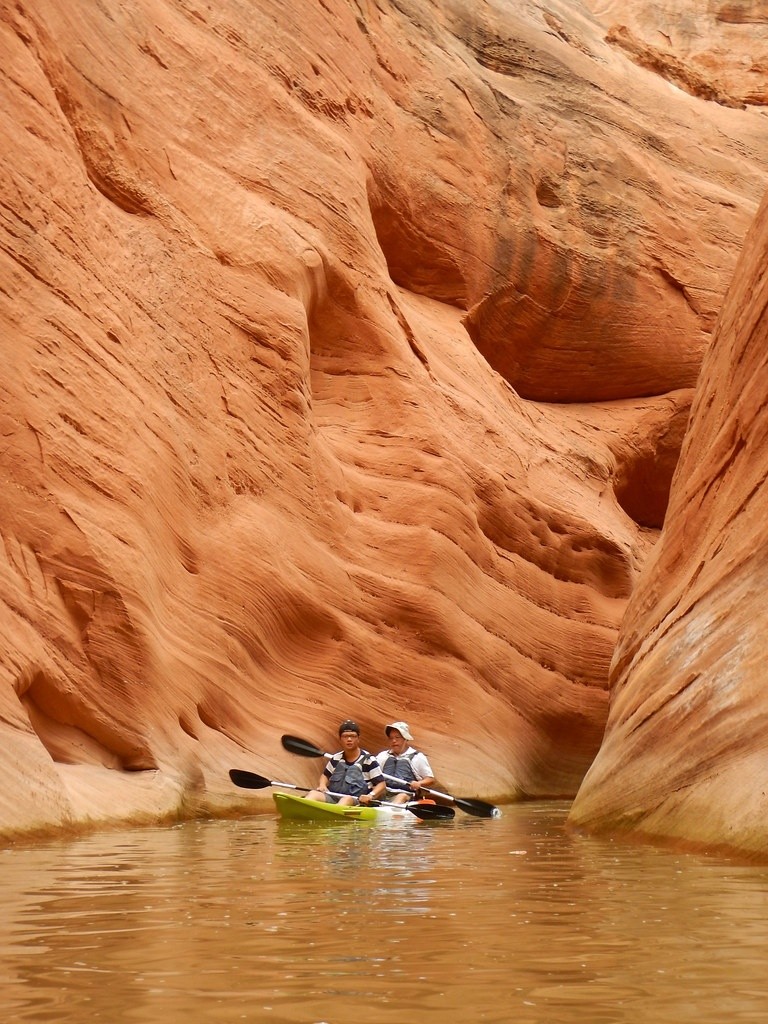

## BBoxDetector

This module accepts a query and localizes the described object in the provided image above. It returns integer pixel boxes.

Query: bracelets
[370,792,376,797]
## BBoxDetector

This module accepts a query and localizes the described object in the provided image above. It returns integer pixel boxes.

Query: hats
[385,722,414,740]
[339,720,359,736]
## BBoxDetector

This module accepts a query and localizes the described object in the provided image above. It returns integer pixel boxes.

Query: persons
[305,721,386,807]
[376,721,435,805]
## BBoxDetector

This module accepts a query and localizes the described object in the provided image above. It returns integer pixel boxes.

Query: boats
[271,790,437,822]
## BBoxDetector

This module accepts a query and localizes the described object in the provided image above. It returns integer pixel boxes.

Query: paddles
[228,768,456,821]
[280,734,503,819]
